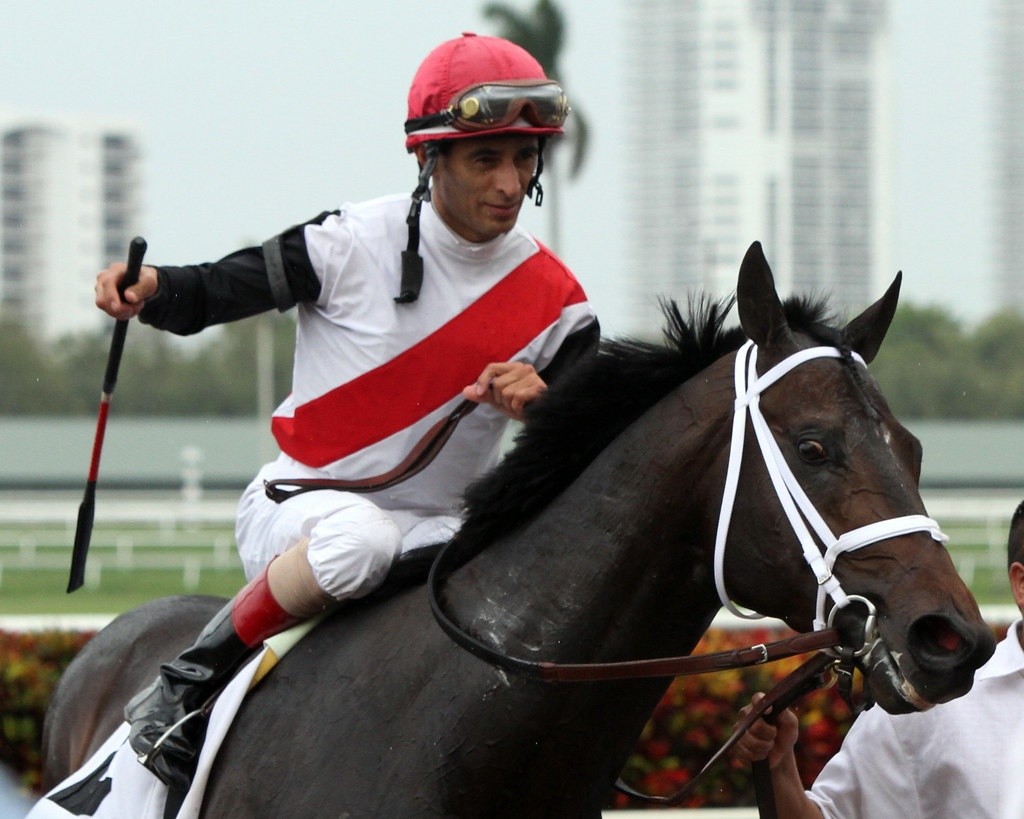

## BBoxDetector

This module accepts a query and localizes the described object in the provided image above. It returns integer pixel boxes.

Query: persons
[97,35,601,790]
[733,501,1024,819]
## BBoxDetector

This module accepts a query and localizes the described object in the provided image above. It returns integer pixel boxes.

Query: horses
[40,239,997,819]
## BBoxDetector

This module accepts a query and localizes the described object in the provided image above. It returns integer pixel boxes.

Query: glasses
[449,79,571,132]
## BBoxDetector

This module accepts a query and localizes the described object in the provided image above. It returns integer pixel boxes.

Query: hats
[404,31,574,155]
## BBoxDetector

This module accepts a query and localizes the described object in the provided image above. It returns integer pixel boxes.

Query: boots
[122,553,307,792]
